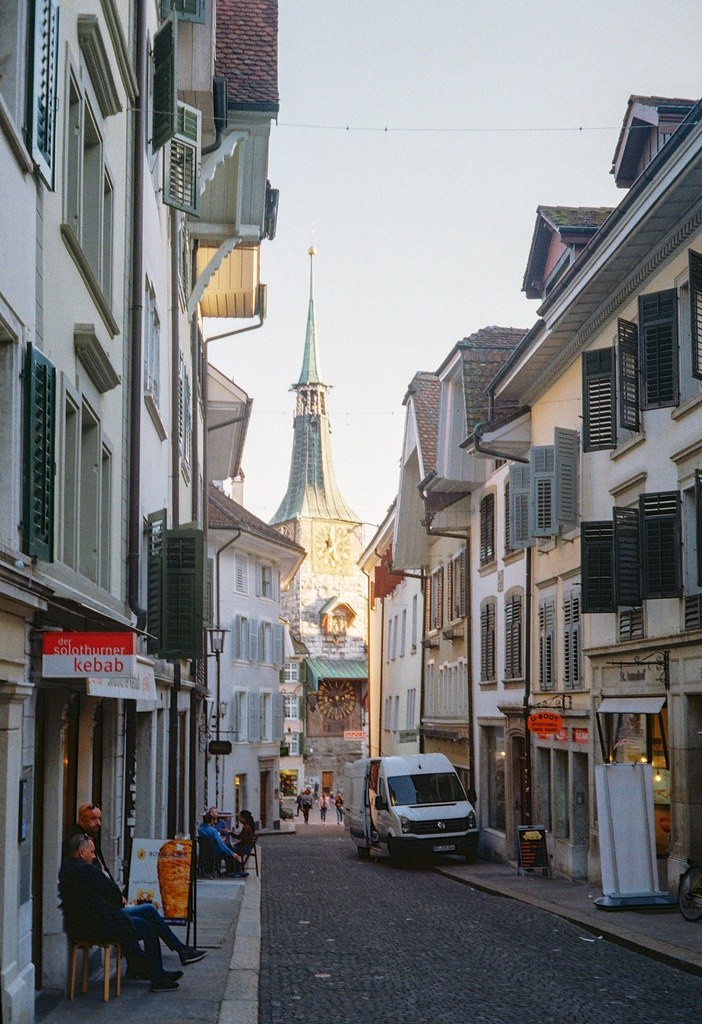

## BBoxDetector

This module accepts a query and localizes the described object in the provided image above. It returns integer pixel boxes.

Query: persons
[279,785,343,825]
[197,806,255,878]
[58,803,208,992]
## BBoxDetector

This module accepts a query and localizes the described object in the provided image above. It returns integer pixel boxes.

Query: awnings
[305,659,368,691]
[595,698,669,771]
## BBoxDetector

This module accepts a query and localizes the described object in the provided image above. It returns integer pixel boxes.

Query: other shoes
[163,970,184,982]
[150,974,180,992]
[179,946,208,966]
[234,870,249,878]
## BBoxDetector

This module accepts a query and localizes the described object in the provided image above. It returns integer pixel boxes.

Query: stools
[70,942,120,1000]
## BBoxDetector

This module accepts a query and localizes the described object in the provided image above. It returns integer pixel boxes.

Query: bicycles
[678,857,702,923]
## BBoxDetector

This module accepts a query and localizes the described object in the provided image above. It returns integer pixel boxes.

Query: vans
[346,753,479,867]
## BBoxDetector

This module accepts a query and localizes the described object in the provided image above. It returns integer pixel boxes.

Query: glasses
[76,833,95,850]
[80,802,101,814]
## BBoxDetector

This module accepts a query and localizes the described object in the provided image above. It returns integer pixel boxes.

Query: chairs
[198,839,222,879]
[240,832,260,876]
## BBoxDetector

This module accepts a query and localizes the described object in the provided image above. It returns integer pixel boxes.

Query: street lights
[209,628,230,807]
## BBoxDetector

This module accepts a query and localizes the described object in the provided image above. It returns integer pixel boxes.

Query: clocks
[318,680,356,720]
[314,524,352,568]
